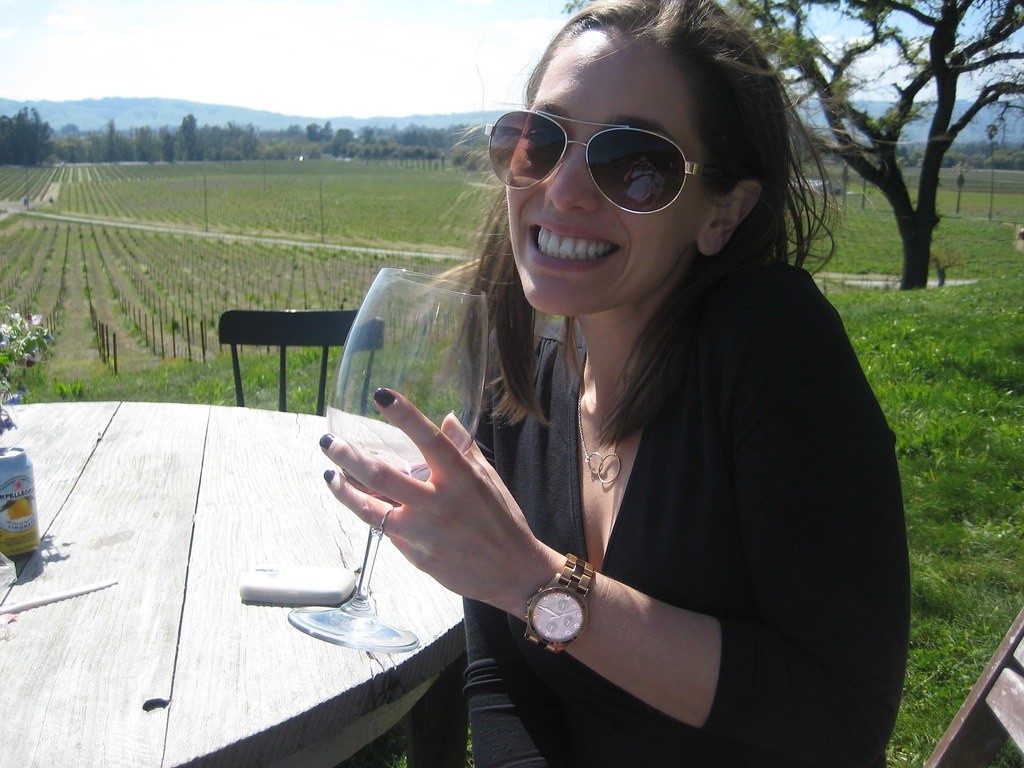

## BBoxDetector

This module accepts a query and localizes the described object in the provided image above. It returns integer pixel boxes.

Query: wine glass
[287,269,487,652]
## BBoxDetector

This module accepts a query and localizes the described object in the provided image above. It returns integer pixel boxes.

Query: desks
[0,400,465,768]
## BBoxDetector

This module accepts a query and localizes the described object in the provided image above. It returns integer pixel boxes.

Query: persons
[320,2,911,768]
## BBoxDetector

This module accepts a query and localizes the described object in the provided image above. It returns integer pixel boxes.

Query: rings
[372,504,394,540]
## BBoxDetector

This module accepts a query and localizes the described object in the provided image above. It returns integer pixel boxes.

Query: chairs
[922,606,1024,768]
[216,310,385,415]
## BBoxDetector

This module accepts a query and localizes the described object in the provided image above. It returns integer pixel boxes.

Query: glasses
[485,109,716,215]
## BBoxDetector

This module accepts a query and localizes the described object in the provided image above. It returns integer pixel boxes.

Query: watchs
[523,553,595,656]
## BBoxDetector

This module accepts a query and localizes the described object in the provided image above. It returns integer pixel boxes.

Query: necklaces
[578,384,622,484]
